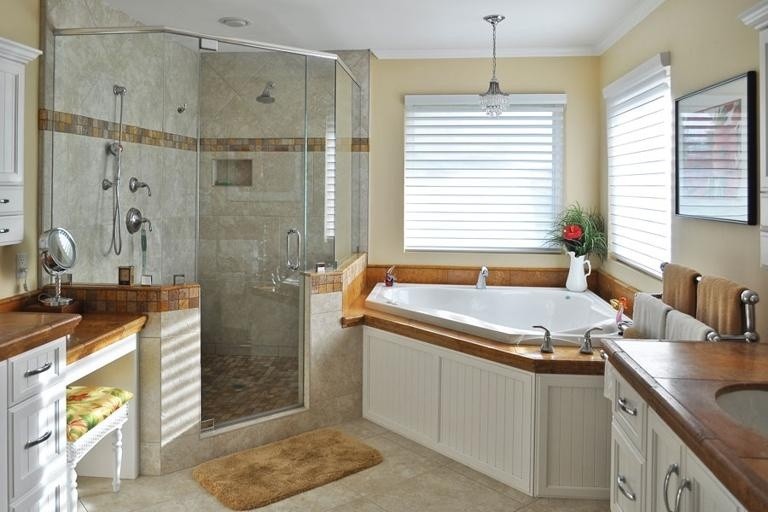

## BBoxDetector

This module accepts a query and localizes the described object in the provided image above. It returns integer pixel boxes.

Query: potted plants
[543,202,607,292]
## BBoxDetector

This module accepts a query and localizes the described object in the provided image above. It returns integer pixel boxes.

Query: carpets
[190,426,382,511]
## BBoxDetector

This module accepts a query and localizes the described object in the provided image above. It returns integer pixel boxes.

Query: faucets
[475,265,489,290]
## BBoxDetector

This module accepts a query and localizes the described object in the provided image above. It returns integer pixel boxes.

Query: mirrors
[38,227,77,307]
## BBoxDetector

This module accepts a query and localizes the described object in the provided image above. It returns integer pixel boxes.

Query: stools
[67,385,133,511]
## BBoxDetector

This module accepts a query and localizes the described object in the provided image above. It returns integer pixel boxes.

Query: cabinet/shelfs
[654,439,738,511]
[8,340,65,403]
[4,216,22,242]
[0,37,44,183]
[6,388,65,498]
[613,384,643,448]
[609,421,649,511]
[0,188,23,213]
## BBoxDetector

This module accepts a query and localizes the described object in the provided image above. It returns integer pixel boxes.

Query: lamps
[479,15,511,116]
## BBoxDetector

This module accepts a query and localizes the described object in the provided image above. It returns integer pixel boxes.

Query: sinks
[716,381,768,438]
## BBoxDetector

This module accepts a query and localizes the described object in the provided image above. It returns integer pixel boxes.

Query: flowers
[563,225,582,240]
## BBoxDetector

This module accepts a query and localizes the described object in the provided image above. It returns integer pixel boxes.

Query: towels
[697,274,751,336]
[632,291,673,341]
[667,308,715,343]
[663,264,701,317]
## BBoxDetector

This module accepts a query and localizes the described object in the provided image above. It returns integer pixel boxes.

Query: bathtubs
[363,280,635,347]
